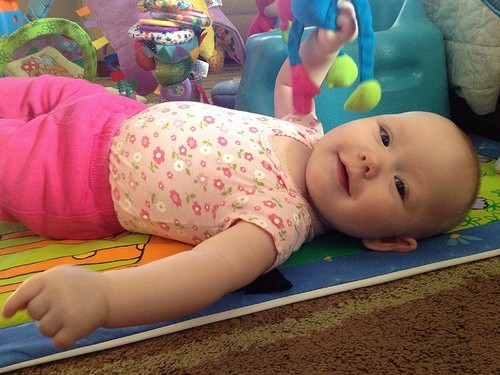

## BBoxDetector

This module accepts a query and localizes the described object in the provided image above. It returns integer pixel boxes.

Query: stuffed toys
[244,0,382,115]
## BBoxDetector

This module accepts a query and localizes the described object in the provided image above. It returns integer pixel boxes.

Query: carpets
[0,254,500,375]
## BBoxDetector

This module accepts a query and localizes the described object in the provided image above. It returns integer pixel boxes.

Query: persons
[1,0,482,350]
